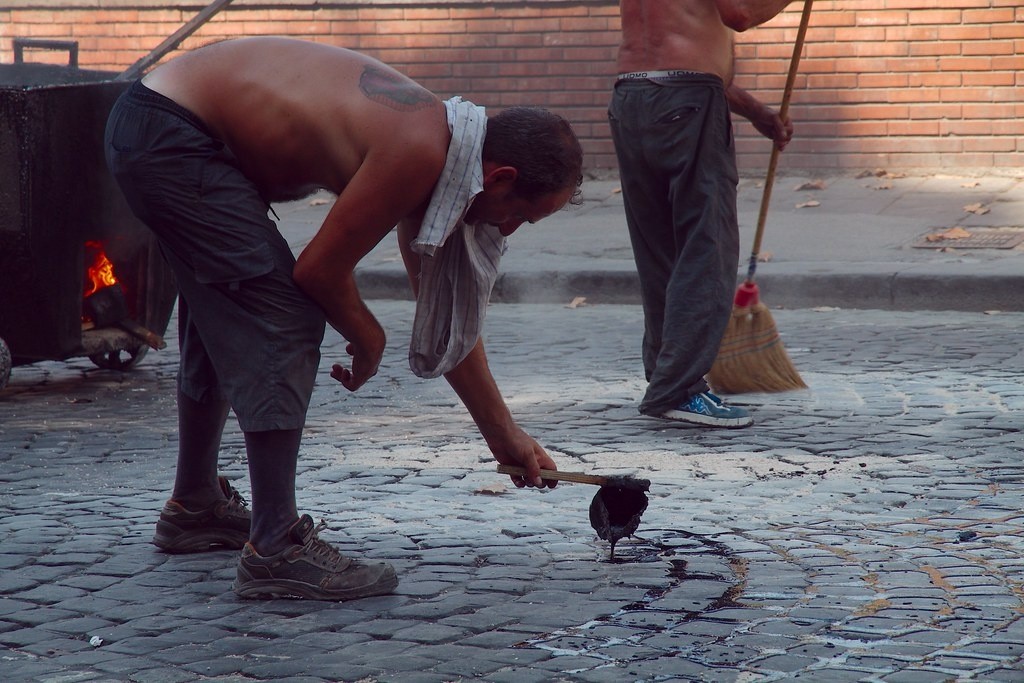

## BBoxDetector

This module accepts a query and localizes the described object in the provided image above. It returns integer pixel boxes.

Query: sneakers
[151,475,257,551]
[660,383,753,429]
[235,511,397,601]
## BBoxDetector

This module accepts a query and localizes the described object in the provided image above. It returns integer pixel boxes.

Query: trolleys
[0,37,199,394]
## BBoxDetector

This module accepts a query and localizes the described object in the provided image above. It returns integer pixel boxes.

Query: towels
[406,96,508,382]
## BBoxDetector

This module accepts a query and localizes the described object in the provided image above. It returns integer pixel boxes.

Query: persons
[606,0,794,429]
[102,33,585,599]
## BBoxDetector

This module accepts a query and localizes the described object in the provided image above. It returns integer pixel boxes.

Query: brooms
[704,0,816,397]
